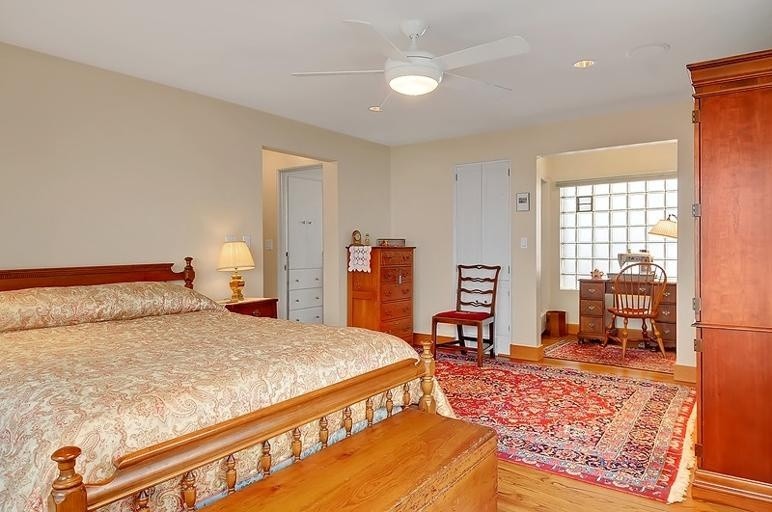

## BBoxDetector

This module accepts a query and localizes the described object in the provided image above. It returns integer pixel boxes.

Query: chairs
[606,261,669,359]
[428,265,501,367]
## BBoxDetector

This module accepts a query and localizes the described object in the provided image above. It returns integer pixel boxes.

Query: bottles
[365,233,370,246]
[628,240,632,253]
[352,224,361,245]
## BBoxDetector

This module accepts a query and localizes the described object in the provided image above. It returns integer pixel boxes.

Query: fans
[274,17,533,116]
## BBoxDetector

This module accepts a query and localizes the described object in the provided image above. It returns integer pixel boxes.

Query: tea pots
[590,269,604,280]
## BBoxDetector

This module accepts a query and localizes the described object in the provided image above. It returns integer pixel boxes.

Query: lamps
[215,241,256,303]
[648,214,678,238]
[383,49,444,98]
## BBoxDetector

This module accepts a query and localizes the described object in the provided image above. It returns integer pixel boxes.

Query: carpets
[412,344,698,504]
[541,334,676,374]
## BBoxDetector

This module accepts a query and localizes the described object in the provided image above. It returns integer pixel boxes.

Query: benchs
[198,406,499,512]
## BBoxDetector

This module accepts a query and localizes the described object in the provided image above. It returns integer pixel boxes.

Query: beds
[1,257,458,512]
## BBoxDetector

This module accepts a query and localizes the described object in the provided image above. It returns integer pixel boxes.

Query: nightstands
[216,296,279,319]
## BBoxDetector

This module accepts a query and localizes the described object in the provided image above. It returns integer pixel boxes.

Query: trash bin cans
[546,311,566,337]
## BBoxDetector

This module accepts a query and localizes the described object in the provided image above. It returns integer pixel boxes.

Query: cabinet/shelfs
[681,49,772,512]
[577,276,678,348]
[346,247,416,346]
